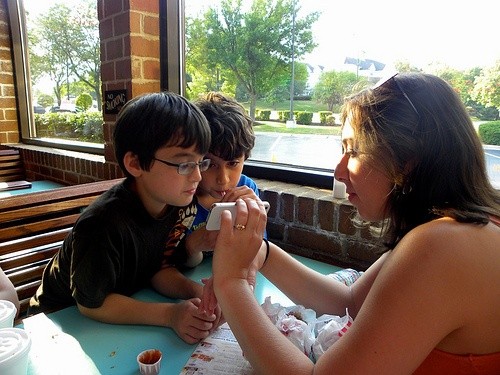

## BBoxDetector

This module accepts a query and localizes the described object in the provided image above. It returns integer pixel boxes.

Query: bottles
[327,268,365,287]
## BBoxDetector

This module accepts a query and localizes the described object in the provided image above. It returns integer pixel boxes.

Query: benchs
[0,150,128,322]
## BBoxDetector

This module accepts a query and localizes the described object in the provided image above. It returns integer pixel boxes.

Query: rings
[233,224,245,230]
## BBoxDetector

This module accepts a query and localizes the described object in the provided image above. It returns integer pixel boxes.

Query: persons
[212,72,500,375]
[0,266,21,318]
[26,92,227,344]
[179,92,267,267]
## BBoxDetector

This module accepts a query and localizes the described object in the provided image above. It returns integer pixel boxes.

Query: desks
[0,180,65,198]
[13,247,366,375]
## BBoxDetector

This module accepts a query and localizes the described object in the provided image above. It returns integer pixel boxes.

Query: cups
[0,299,17,328]
[0,327,32,375]
[333,177,346,198]
[136,349,162,375]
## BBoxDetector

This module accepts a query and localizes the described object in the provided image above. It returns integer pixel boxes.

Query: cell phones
[205,201,270,230]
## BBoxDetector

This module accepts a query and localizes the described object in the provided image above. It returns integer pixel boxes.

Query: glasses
[151,156,211,176]
[373,70,420,117]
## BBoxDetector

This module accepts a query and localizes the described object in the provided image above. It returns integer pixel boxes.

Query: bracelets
[263,238,270,263]
